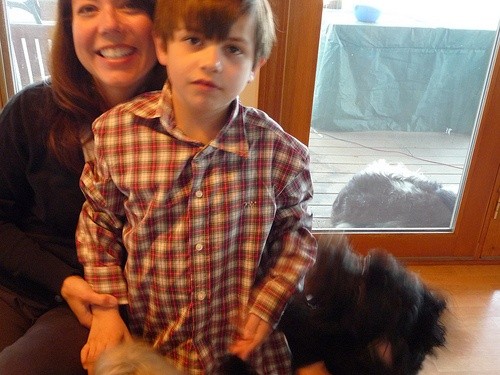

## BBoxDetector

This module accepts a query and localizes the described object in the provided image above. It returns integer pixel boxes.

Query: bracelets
[53,270,86,306]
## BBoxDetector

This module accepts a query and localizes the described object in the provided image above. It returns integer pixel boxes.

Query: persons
[75,0,317,375]
[1,1,330,375]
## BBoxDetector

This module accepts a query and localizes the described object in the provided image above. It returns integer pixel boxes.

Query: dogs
[217,234,453,375]
[331,160,458,228]
